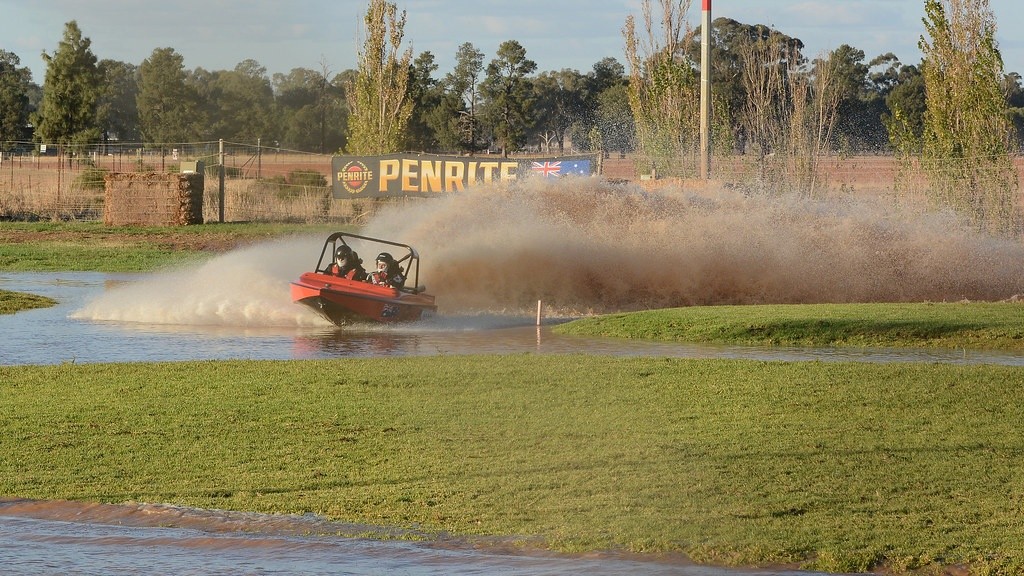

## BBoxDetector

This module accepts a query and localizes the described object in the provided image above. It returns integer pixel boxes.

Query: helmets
[376,253,394,264]
[334,245,353,259]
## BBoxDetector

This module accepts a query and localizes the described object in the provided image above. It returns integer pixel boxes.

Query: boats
[289,231,440,327]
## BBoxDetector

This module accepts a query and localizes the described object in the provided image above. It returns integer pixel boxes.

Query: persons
[323,245,366,283]
[366,252,405,290]
